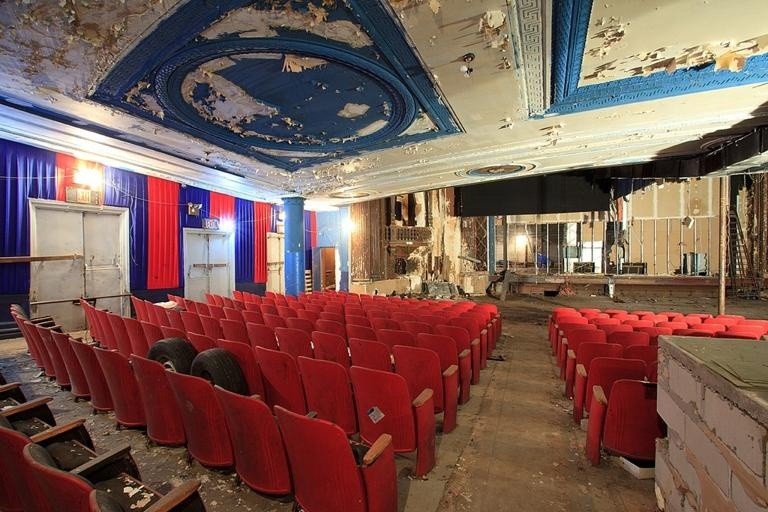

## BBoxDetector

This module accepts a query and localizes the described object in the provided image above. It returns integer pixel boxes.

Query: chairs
[548,307,768,467]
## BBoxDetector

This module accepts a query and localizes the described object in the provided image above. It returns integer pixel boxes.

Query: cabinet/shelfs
[312,245,336,294]
